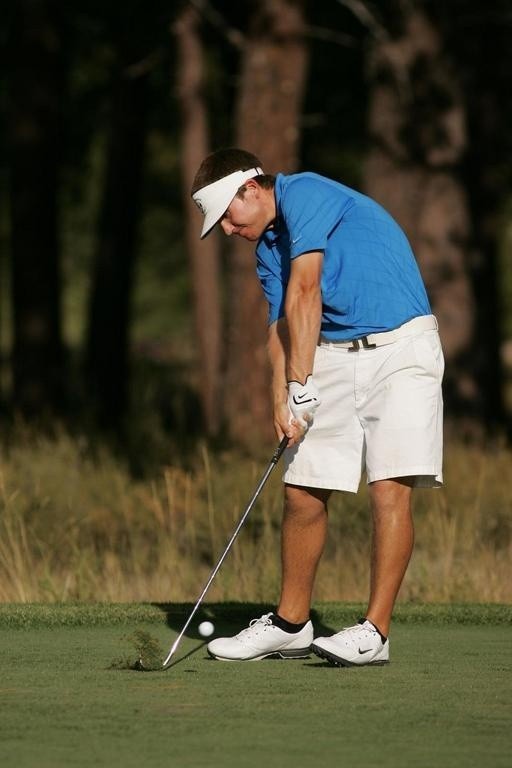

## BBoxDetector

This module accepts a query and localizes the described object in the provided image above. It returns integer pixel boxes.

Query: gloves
[287,374,322,433]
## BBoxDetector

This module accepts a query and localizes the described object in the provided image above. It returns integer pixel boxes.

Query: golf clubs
[198,622,214,637]
[136,435,289,670]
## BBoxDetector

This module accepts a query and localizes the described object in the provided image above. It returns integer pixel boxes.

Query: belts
[320,316,436,351]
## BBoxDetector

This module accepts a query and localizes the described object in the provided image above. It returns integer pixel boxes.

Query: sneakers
[311,620,390,666]
[206,612,315,662]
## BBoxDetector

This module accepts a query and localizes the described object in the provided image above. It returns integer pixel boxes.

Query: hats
[192,166,265,240]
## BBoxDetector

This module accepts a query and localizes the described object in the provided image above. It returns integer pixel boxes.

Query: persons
[191,146,445,668]
